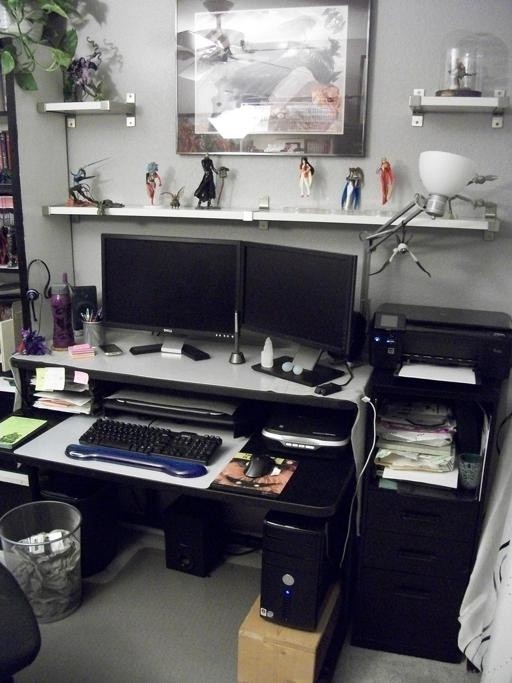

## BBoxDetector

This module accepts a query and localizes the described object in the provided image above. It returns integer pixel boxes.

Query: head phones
[25,258,53,301]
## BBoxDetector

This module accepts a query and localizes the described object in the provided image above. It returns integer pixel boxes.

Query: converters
[316,382,343,396]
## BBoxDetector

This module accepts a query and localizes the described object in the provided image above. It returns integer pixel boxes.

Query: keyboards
[78,418,223,466]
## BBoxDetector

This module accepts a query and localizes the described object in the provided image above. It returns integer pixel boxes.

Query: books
[1,74,17,268]
[0,415,48,449]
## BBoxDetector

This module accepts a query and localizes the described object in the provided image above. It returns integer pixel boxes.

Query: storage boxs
[237,589,341,683]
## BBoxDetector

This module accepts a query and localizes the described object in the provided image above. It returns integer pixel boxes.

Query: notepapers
[68,344,96,358]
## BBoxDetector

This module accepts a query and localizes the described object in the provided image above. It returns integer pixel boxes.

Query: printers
[361,303,512,408]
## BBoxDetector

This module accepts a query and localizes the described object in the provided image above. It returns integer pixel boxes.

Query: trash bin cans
[0,501,82,625]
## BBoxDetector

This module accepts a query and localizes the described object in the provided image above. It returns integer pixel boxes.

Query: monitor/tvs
[100,233,241,361]
[237,241,357,387]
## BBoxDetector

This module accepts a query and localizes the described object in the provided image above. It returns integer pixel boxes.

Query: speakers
[71,284,98,331]
[162,494,227,578]
[327,310,367,364]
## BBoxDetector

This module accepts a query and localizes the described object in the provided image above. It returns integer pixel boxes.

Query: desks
[0,322,376,580]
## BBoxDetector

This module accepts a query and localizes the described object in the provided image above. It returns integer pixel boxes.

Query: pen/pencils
[81,306,105,347]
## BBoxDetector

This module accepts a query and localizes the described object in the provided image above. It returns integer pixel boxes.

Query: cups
[458,453,484,490]
[50,284,75,349]
[82,321,106,348]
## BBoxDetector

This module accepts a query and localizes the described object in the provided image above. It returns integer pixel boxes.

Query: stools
[0,563,42,682]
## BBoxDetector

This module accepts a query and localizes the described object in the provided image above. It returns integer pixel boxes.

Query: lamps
[329,149,478,369]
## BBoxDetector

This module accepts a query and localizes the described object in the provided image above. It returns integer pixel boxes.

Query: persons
[145,162,162,203]
[297,155,314,198]
[193,155,230,208]
[68,158,109,205]
[376,158,396,207]
[341,167,364,210]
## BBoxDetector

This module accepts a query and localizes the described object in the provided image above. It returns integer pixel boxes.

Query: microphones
[32,300,37,322]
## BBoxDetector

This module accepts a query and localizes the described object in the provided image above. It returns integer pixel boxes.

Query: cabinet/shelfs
[0,37,76,513]
[348,476,484,664]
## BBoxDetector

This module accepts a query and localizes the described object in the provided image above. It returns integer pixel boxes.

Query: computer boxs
[258,508,348,633]
[37,472,125,579]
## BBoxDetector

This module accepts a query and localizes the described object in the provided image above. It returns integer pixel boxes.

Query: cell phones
[97,344,123,356]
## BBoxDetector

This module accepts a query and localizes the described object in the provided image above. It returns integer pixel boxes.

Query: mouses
[244,454,276,479]
